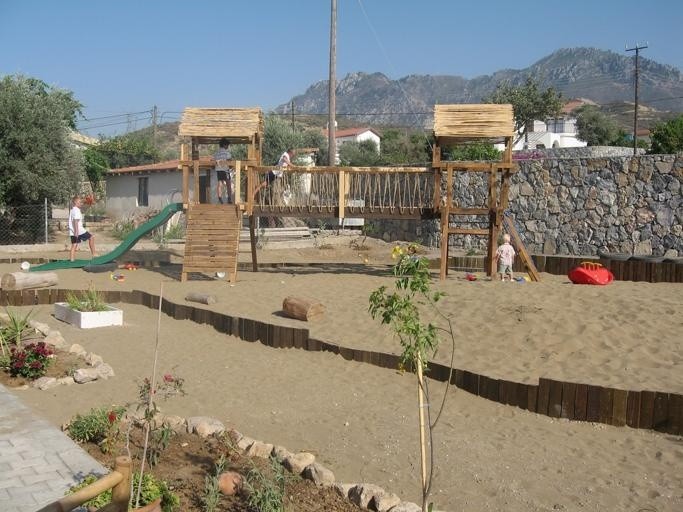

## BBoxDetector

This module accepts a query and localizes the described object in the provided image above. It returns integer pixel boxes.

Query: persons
[494,233,516,281]
[253,148,296,201]
[209,138,233,204]
[66,196,99,262]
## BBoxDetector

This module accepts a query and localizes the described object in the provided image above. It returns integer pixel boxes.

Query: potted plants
[51,283,125,329]
[95,207,107,222]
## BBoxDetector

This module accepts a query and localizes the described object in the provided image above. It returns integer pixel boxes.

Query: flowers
[85,194,97,216]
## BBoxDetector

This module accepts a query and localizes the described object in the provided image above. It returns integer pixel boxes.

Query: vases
[84,215,95,222]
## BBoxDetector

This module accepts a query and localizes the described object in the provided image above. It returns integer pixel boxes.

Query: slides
[28,203,183,272]
